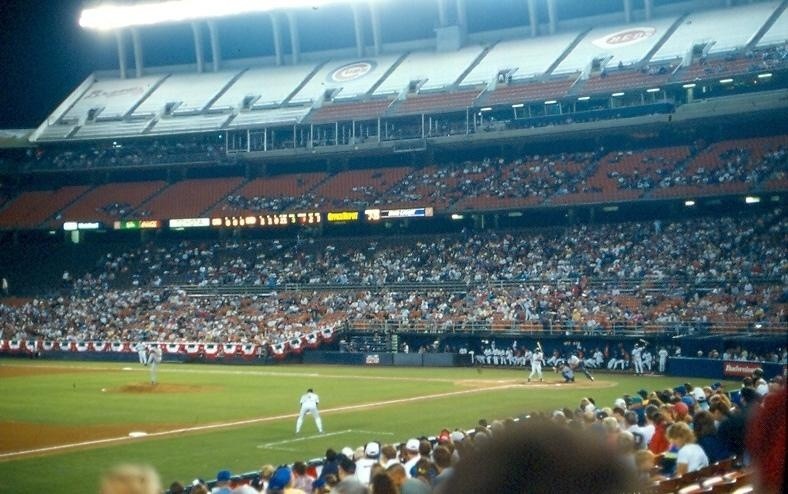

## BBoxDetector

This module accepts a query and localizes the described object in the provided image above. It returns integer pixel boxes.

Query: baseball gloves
[553,366,560,373]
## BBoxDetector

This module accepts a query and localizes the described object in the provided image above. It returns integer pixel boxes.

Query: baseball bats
[639,338,650,344]
[537,342,542,353]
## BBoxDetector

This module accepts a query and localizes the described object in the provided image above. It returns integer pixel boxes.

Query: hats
[365,442,380,457]
[268,466,291,490]
[406,439,420,452]
[217,470,230,481]
